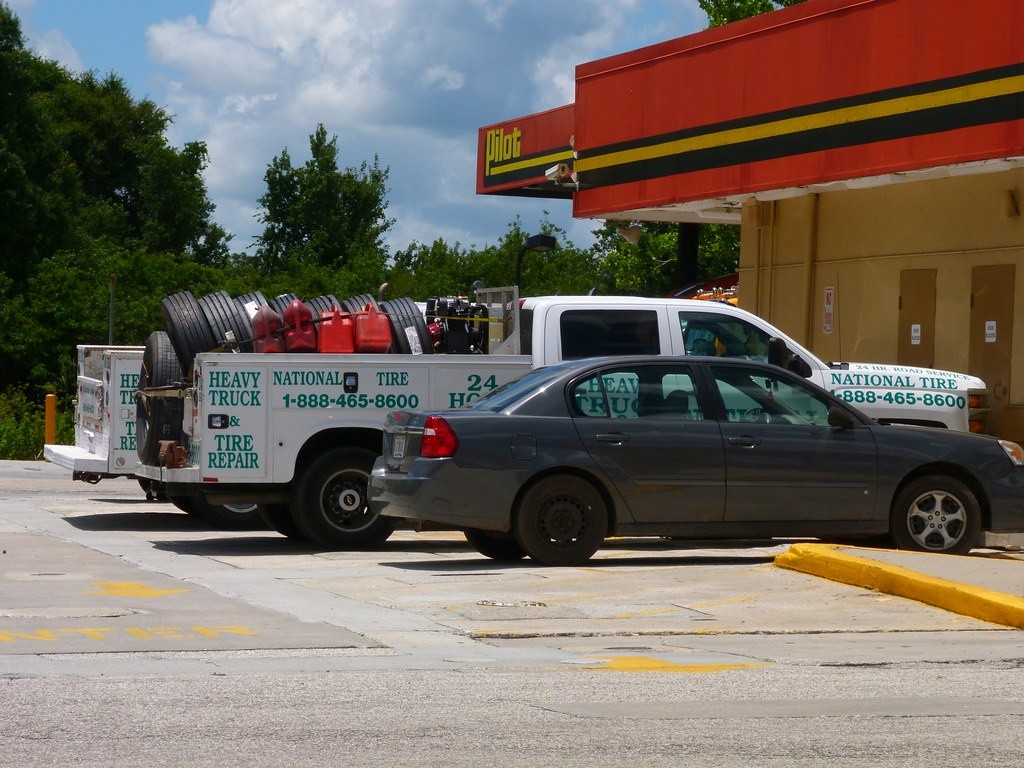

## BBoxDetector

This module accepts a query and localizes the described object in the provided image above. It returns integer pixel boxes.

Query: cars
[367,356,1024,566]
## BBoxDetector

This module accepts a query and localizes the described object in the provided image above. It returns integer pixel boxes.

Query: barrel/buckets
[251,299,393,354]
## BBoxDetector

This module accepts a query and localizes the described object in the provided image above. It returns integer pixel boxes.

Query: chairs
[667,389,689,421]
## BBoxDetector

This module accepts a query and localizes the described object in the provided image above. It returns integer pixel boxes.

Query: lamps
[615,218,642,245]
[998,189,1018,220]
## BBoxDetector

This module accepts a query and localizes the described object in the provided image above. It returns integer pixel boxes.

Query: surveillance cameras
[545,163,569,181]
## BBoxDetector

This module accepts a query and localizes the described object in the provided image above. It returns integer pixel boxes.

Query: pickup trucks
[44,287,993,552]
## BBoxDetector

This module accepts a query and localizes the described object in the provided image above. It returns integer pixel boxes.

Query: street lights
[514,234,556,298]
